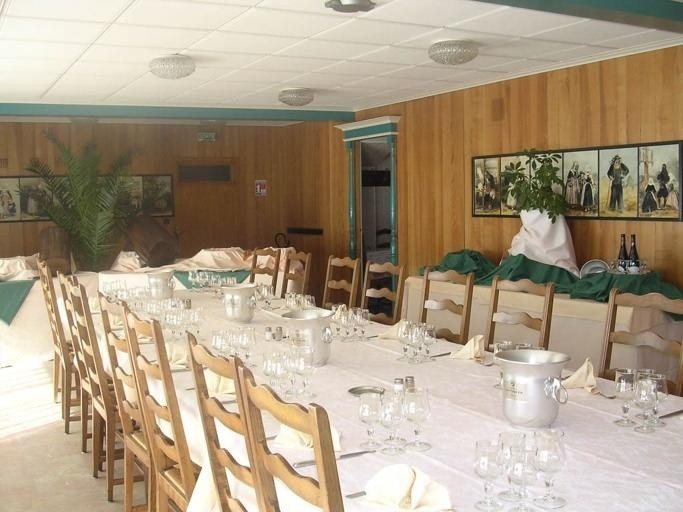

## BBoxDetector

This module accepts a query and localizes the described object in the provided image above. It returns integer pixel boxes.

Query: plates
[580,258,608,279]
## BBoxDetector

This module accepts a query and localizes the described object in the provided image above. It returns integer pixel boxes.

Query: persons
[483,171,496,210]
[606,154,629,212]
[640,161,679,214]
[564,161,595,214]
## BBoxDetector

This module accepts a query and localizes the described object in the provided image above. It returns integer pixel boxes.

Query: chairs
[250,248,407,324]
[96,291,345,512]
[484,276,556,354]
[597,288,683,397]
[35,260,123,500]
[417,266,475,345]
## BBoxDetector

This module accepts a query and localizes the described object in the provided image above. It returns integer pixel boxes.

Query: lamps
[426,38,478,64]
[277,88,313,106]
[148,54,196,79]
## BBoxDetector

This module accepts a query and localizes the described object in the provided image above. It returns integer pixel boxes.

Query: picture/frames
[0,171,175,224]
[468,138,683,222]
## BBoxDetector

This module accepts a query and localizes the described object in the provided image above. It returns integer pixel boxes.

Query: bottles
[616,234,628,274]
[629,234,640,276]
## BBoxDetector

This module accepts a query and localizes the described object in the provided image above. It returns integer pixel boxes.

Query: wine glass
[358,322,671,511]
[103,269,371,398]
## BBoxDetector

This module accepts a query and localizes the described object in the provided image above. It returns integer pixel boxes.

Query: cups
[609,260,653,276]
